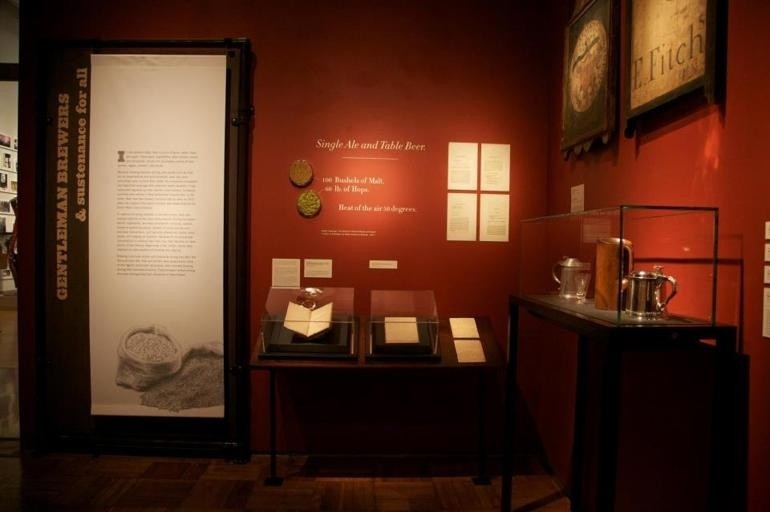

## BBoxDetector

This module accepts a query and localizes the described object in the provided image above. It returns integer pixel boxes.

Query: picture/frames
[559,1,716,156]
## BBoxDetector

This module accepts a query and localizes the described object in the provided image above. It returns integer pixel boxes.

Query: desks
[251,316,505,487]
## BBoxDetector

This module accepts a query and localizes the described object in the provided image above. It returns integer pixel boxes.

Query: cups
[552,237,678,321]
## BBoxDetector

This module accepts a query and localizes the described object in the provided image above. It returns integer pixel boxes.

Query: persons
[9,216,18,288]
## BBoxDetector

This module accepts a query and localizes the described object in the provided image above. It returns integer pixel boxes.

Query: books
[283,301,334,339]
